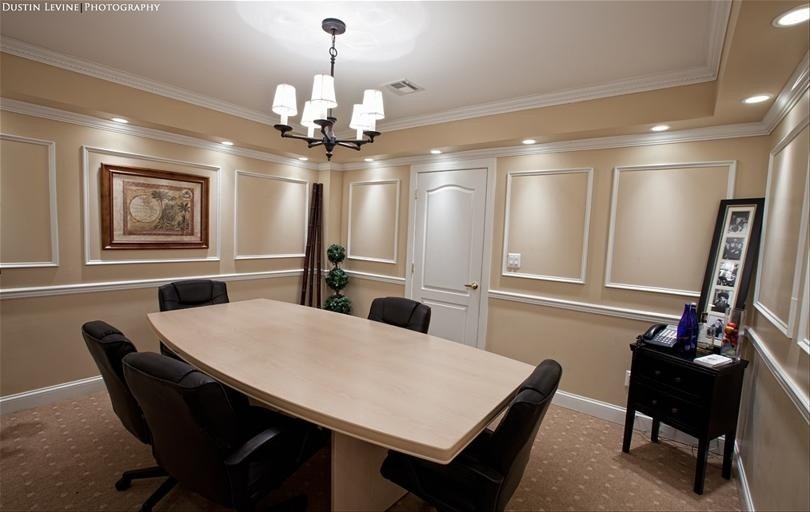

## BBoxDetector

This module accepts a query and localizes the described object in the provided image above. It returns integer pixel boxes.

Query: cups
[721,308,747,361]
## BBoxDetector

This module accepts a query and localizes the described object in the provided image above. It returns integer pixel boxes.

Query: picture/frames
[697,198,765,355]
[99,162,212,250]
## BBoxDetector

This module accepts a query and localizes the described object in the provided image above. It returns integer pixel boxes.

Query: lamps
[271,17,386,161]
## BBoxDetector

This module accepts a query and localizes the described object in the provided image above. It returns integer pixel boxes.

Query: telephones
[641,323,678,349]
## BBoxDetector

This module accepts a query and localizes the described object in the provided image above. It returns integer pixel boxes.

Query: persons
[713,292,729,312]
[709,318,722,336]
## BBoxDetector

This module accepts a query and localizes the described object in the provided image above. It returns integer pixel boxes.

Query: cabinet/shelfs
[622,342,749,495]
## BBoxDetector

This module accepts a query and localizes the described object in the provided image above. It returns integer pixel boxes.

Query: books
[693,353,733,368]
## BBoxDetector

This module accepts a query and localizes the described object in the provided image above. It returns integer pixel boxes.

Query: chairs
[82,321,177,512]
[158,281,229,364]
[121,351,327,512]
[368,297,432,333]
[381,359,563,512]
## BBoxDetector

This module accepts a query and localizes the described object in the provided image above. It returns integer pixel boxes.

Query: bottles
[677,303,692,350]
[699,312,714,349]
[690,303,699,349]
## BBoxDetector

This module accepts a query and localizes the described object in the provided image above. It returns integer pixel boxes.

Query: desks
[147,298,537,511]
